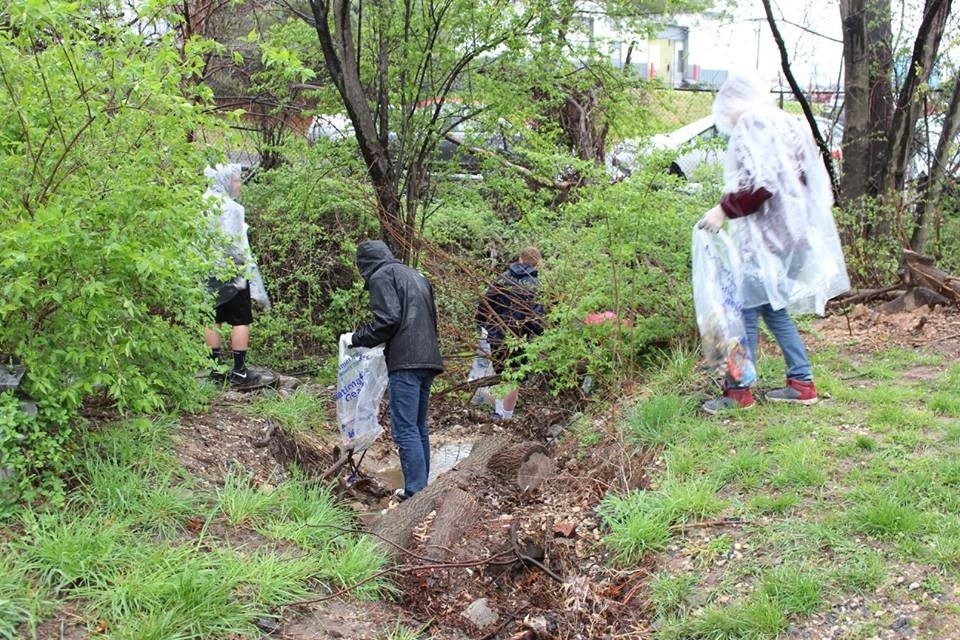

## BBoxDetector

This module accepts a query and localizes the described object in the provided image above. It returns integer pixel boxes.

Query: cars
[811,89,819,102]
[917,94,938,117]
[612,112,842,182]
[306,104,401,148]
[905,114,960,181]
[830,93,845,108]
[389,103,525,174]
[817,93,832,103]
[415,98,540,131]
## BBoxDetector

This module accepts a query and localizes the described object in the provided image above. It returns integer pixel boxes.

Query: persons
[340,239,446,502]
[703,54,852,416]
[189,160,272,389]
[466,246,546,418]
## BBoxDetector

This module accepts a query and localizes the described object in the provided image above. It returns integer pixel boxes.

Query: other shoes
[230,367,277,389]
[393,488,410,502]
[702,388,758,416]
[764,379,820,406]
